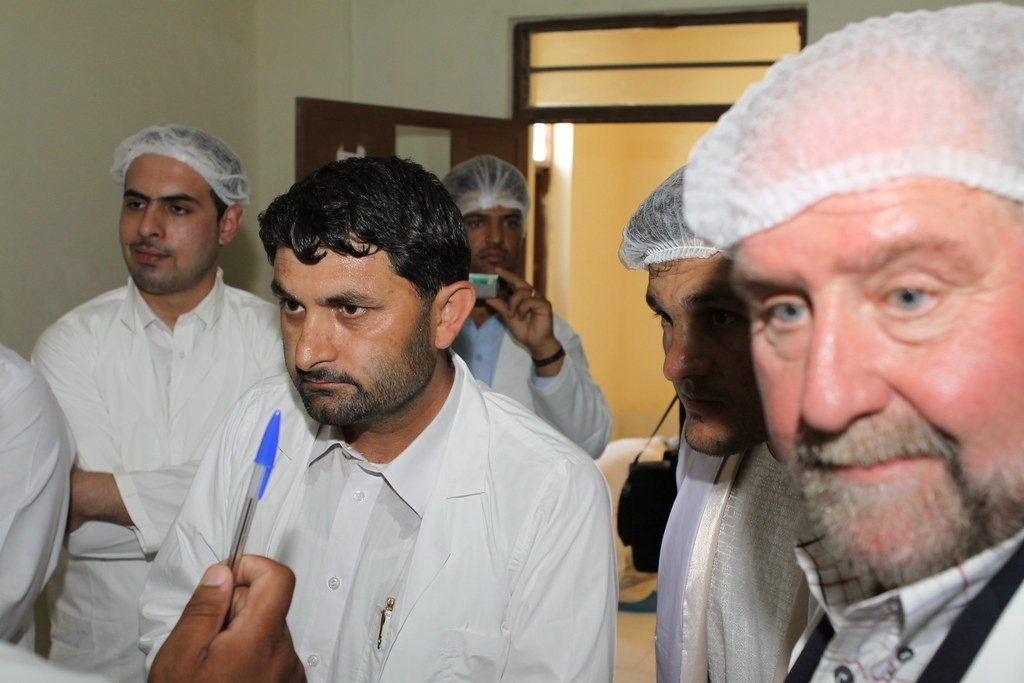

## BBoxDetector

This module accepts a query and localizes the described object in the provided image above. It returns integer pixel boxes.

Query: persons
[0,122,818,683]
[680,3,1024,682]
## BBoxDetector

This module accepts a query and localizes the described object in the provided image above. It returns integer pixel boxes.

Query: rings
[530,287,537,299]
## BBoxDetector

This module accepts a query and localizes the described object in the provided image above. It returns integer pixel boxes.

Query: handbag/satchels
[617,461,678,573]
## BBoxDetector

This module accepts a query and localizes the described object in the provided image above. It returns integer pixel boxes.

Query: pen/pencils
[225,409,283,572]
[377,597,396,649]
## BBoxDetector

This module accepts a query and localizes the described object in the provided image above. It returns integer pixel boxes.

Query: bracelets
[532,347,565,368]
